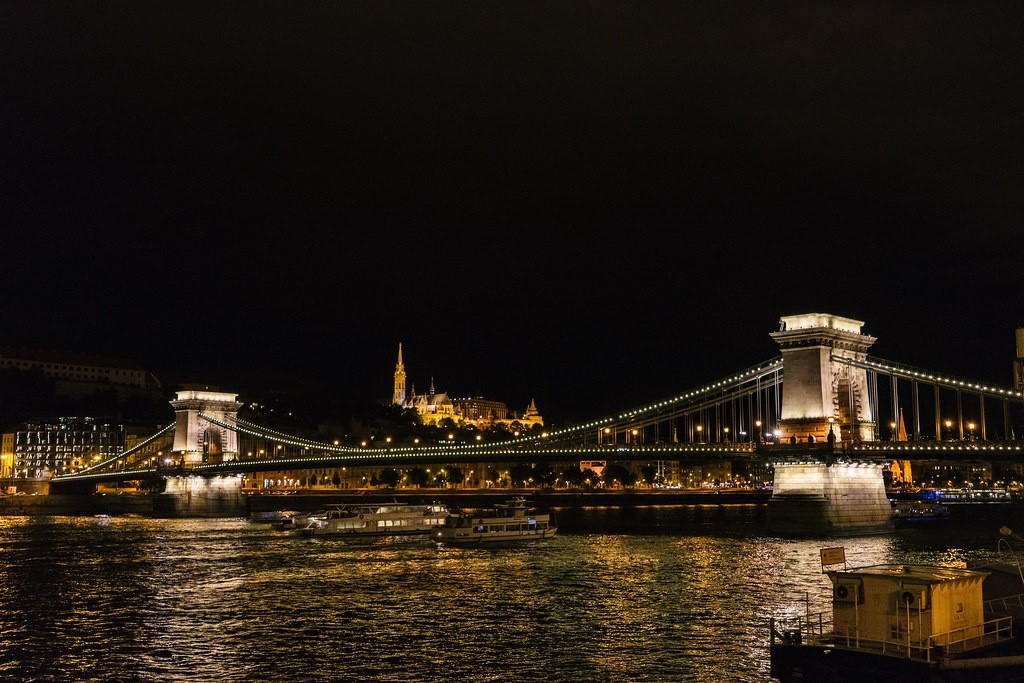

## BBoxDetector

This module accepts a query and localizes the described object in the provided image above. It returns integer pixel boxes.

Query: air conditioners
[897,588,926,609]
[834,583,859,603]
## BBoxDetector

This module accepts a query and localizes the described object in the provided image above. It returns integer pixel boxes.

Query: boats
[272,509,359,531]
[917,488,1015,505]
[889,499,950,518]
[429,496,558,542]
[769,524,1024,682]
[303,505,451,537]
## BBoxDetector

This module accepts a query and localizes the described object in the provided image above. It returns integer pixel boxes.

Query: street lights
[386,437,391,454]
[248,451,252,462]
[477,435,481,451]
[158,451,162,467]
[362,442,367,450]
[204,441,208,459]
[181,450,185,468]
[633,430,639,446]
[277,445,282,460]
[449,434,453,452]
[415,439,419,454]
[723,427,730,444]
[946,421,952,440]
[697,425,705,444]
[828,417,836,448]
[119,460,122,468]
[522,433,525,453]
[260,449,264,461]
[605,428,610,445]
[95,455,99,471]
[891,423,896,441]
[514,431,519,451]
[334,441,339,446]
[968,422,976,442]
[542,433,547,449]
[755,421,765,447]
